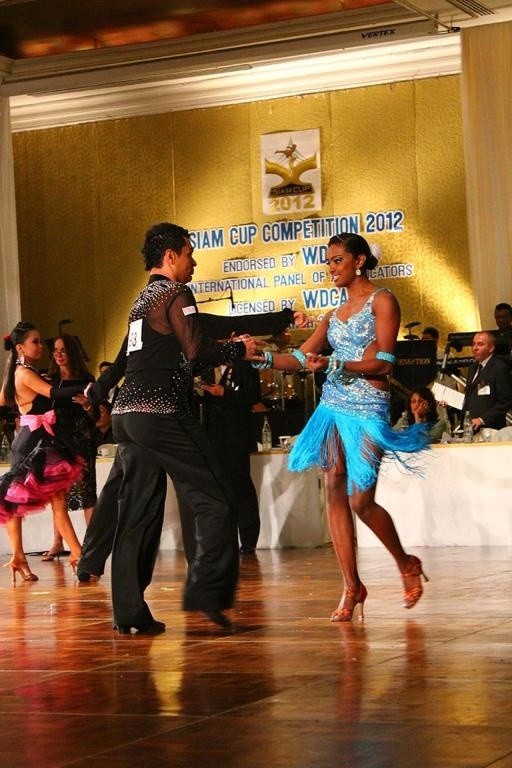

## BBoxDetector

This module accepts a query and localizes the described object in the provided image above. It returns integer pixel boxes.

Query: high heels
[69,557,80,574]
[39,545,64,562]
[398,554,430,608]
[329,585,368,626]
[8,562,38,582]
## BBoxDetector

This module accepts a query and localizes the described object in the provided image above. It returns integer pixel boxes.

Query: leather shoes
[203,609,231,628]
[239,537,256,554]
[114,618,167,635]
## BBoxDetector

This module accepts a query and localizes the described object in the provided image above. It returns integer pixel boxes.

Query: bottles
[262,416,271,451]
[463,410,474,437]
[400,412,409,432]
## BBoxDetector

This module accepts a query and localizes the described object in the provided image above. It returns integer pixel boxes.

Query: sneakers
[77,561,91,584]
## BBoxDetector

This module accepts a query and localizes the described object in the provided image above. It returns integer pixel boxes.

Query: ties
[478,364,483,373]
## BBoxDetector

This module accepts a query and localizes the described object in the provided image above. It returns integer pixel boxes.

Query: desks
[0,439,512,553]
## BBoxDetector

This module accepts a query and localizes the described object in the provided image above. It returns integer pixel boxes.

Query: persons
[0,223,321,633]
[248,233,429,622]
[393,303,510,445]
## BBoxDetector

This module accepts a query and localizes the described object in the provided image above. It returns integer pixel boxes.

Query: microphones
[230,291,236,311]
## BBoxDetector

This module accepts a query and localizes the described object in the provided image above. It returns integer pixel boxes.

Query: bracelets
[324,356,345,376]
[376,352,396,365]
